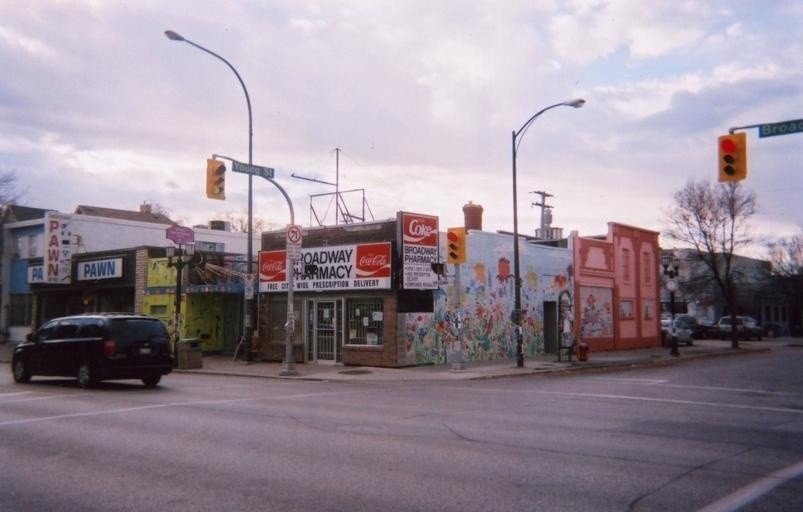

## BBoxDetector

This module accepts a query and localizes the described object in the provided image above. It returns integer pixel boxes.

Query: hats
[661,309,765,347]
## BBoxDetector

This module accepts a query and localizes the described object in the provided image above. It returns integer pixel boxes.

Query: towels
[176,338,204,369]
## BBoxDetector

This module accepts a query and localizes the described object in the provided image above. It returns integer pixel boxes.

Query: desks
[511,98,586,369]
[162,31,254,364]
[166,237,195,368]
[661,252,680,356]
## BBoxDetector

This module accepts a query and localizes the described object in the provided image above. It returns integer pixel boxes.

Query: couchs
[205,160,225,200]
[718,135,744,179]
[446,226,465,264]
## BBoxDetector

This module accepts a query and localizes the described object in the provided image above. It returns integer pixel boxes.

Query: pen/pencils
[9,313,172,388]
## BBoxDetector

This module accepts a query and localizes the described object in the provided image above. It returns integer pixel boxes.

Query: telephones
[759,118,803,138]
[233,162,273,178]
